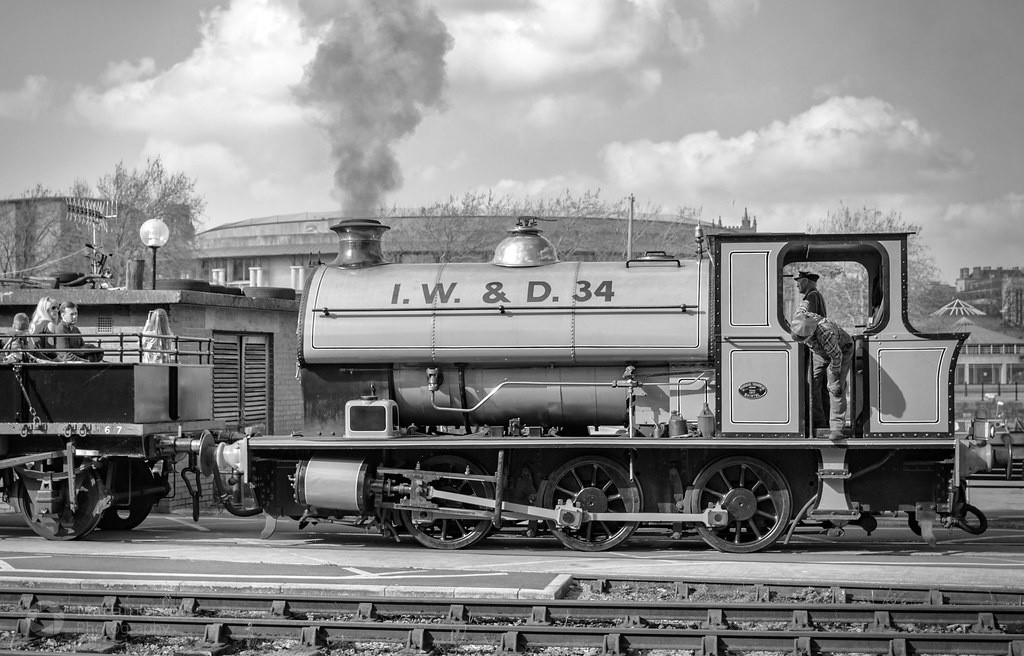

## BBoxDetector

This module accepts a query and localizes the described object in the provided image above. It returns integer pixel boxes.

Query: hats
[791,314,817,342]
[793,270,820,283]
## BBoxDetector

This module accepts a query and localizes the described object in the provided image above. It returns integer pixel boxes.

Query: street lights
[139,218,170,291]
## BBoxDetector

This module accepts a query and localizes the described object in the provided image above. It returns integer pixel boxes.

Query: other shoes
[829,430,843,440]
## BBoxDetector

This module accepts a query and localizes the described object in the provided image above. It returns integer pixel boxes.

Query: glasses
[48,306,59,310]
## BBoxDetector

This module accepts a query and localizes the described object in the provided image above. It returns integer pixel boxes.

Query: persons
[141,309,173,364]
[0,297,109,364]
[793,270,827,426]
[790,312,855,440]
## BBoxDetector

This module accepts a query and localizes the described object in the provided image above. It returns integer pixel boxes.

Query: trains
[0,211,1012,556]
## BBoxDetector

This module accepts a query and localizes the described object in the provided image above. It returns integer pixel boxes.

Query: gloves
[827,380,843,397]
